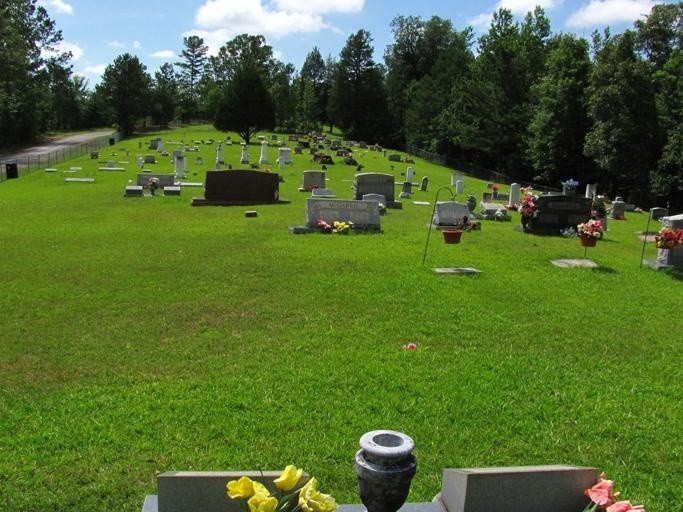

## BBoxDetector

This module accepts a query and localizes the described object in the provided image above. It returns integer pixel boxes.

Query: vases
[442,228,463,244]
[580,237,597,247]
[562,189,578,196]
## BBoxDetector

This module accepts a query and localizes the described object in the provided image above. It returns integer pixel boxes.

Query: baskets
[441,229,462,244]
[579,233,597,247]
[655,235,671,249]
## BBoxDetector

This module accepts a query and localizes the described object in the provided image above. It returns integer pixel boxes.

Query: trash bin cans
[6,164,17,179]
[110,138,114,145]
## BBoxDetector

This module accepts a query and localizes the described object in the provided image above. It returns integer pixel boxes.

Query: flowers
[562,177,580,188]
[223,463,338,512]
[654,227,683,248]
[577,219,605,236]
[317,217,350,233]
[580,470,646,512]
